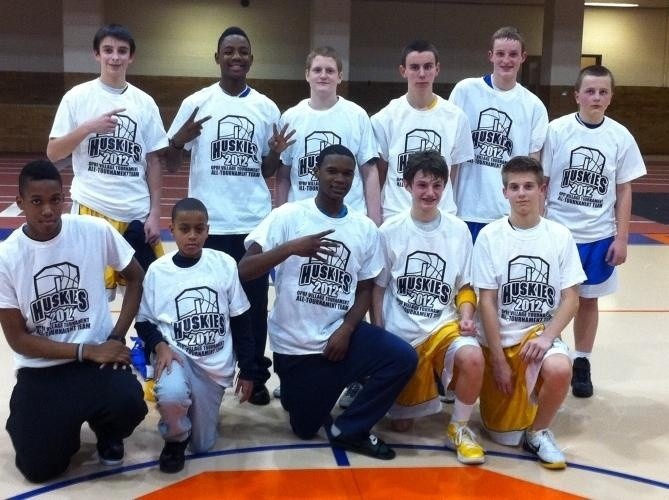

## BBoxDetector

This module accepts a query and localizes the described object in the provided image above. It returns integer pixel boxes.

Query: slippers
[332,432,395,460]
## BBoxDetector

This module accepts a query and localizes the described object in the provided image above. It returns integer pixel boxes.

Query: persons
[469,155,588,470]
[539,63,649,398]
[168,25,297,407]
[135,197,261,476]
[235,143,419,460]
[272,45,383,410]
[0,158,149,485]
[447,24,550,247]
[368,37,476,403]
[371,149,488,465]
[47,21,170,405]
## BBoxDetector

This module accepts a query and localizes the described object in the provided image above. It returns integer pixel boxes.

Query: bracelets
[106,335,127,345]
[76,343,84,363]
[171,137,185,151]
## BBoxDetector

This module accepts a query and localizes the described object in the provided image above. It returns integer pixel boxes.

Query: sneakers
[96,440,124,466]
[571,357,593,397]
[249,384,281,405]
[447,418,486,464]
[160,436,191,473]
[339,384,363,407]
[523,428,567,469]
[438,381,455,401]
